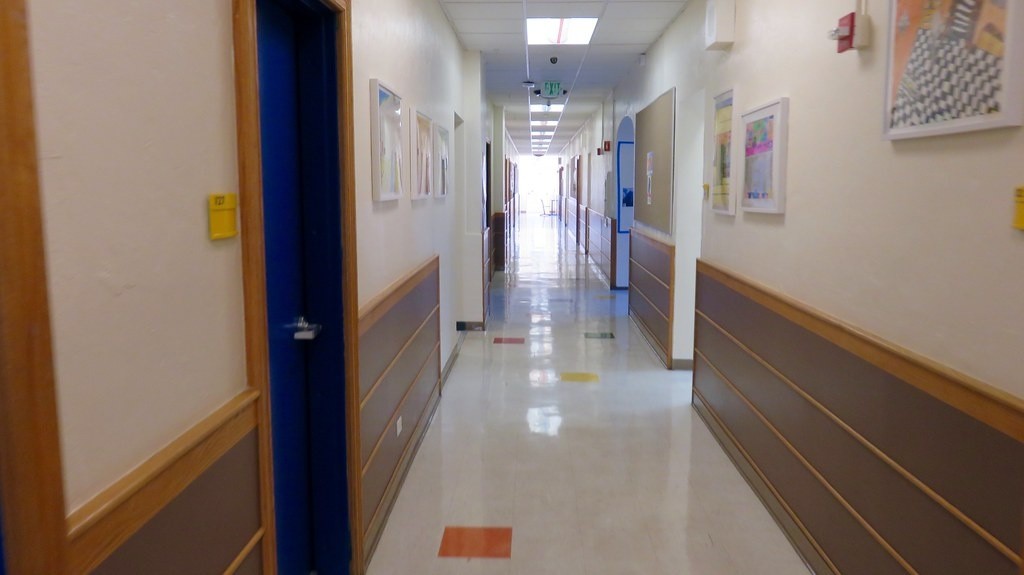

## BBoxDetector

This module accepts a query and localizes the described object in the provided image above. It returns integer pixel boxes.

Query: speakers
[705,0,736,50]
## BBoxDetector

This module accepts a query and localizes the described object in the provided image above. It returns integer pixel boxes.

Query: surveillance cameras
[550,57,557,64]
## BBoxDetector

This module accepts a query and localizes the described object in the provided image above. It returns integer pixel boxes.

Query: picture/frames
[409,105,434,200]
[369,78,406,202]
[432,122,451,199]
[708,86,743,216]
[737,97,790,214]
[879,0,1024,141]
